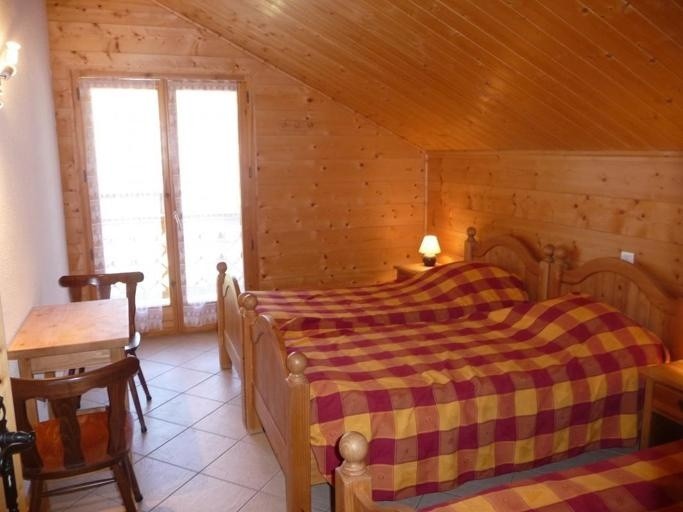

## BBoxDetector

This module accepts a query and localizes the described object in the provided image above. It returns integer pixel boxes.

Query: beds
[209,223,555,446]
[331,411,683,511]
[237,245,683,512]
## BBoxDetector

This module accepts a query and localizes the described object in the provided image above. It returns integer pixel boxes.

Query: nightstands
[635,353,682,451]
[391,261,446,287]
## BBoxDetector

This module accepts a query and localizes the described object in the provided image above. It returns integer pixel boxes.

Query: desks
[2,295,134,468]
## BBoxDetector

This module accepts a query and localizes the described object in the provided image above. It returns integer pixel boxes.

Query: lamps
[417,233,441,266]
[0,40,21,109]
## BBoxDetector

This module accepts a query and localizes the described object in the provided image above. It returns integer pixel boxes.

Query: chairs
[6,354,145,511]
[54,270,153,434]
